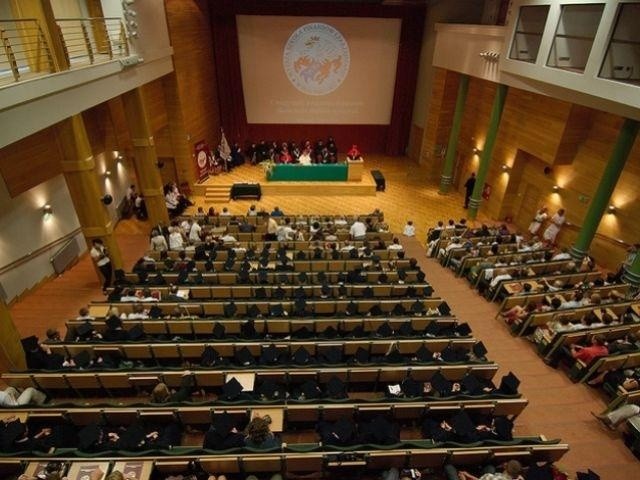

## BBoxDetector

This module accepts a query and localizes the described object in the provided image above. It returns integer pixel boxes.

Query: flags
[219,127,232,162]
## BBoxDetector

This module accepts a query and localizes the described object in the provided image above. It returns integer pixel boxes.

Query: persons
[0,173,640,480]
[230,137,338,168]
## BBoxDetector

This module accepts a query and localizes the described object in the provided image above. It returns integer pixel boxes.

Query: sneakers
[585,379,598,389]
[591,411,617,432]
[543,357,558,369]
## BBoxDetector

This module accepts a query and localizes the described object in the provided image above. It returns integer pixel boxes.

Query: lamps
[44,205,53,214]
[552,185,559,193]
[104,168,113,180]
[608,206,616,215]
[118,154,124,162]
[501,164,508,172]
[472,147,478,154]
